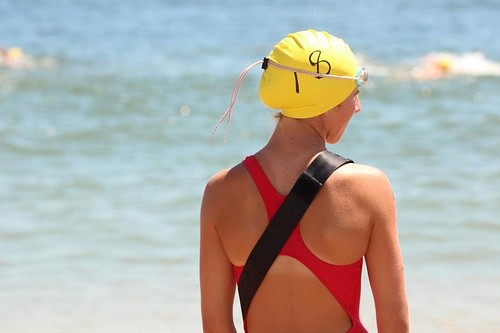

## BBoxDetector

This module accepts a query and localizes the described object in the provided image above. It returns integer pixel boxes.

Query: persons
[197,27,415,333]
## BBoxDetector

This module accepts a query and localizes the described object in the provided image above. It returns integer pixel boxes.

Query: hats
[259,28,361,119]
